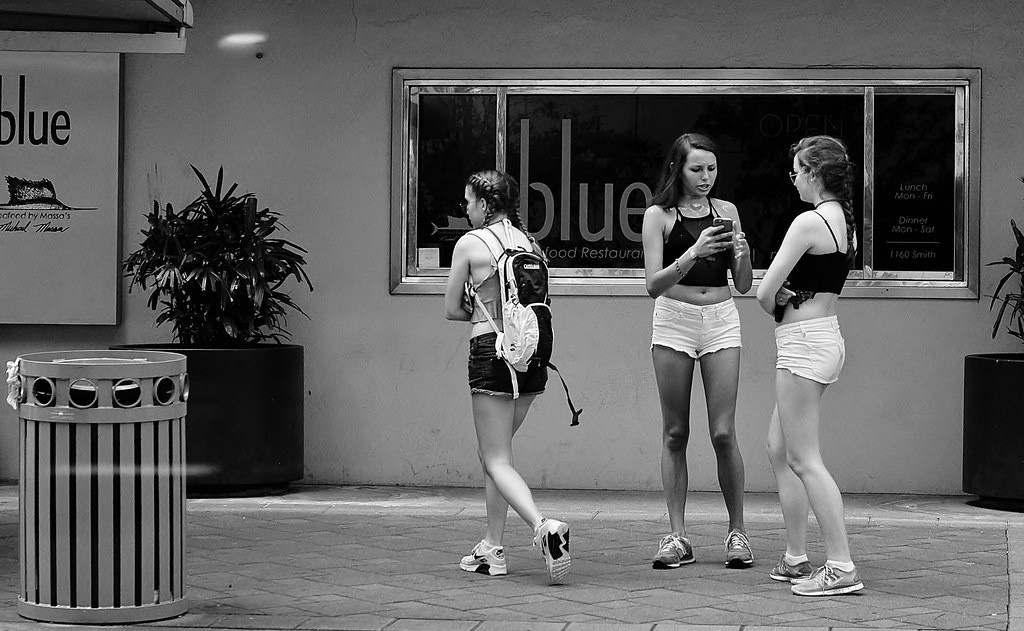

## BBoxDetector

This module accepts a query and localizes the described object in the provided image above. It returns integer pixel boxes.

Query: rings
[779,297,782,302]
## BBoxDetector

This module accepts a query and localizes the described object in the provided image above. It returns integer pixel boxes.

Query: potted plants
[964,221,1024,513]
[109,163,306,495]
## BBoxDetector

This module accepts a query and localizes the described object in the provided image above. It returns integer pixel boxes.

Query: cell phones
[713,218,733,248]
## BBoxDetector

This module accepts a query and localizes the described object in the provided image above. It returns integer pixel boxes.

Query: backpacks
[463,230,554,373]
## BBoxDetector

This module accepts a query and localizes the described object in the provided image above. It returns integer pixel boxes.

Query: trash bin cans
[19,351,190,623]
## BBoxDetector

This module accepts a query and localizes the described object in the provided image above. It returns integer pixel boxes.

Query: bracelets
[687,247,699,261]
[675,258,687,277]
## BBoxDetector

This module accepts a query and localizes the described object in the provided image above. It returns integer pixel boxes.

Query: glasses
[789,169,810,183]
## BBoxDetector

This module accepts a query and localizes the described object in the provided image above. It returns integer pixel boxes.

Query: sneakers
[770,555,815,584]
[652,532,696,569]
[723,529,755,568]
[791,563,864,596]
[459,539,507,576]
[533,519,571,582]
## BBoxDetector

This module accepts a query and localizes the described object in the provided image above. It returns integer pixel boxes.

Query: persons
[757,134,864,595]
[443,171,571,584]
[641,133,754,571]
